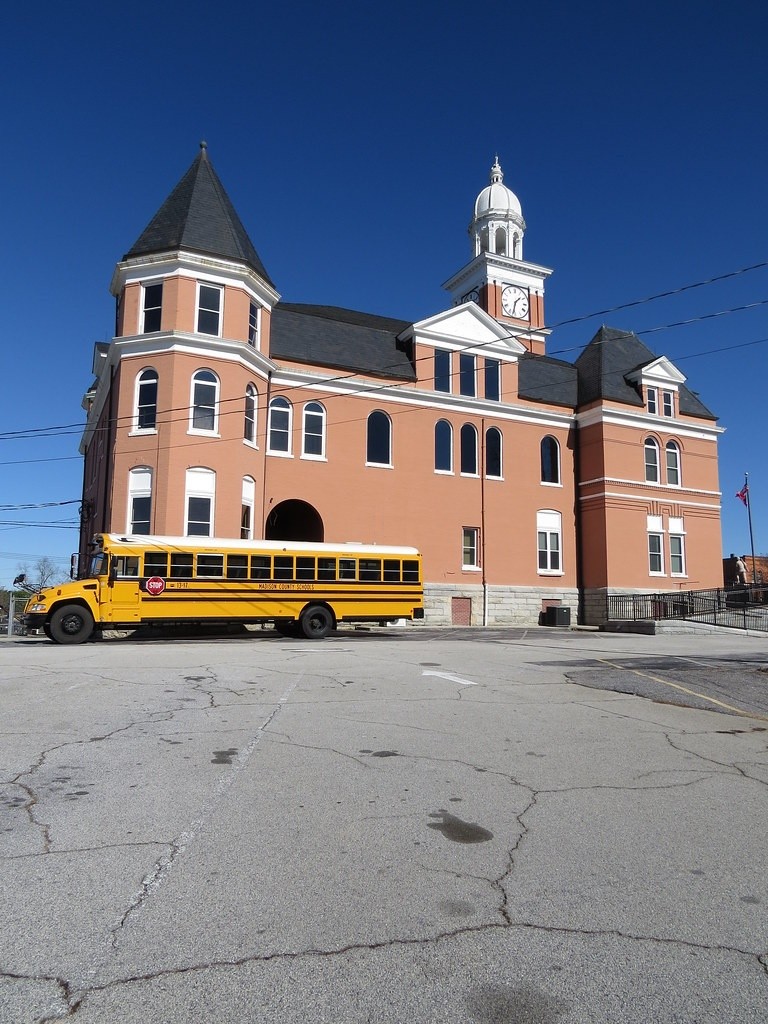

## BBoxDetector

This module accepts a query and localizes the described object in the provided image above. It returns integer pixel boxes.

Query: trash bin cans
[546,606,571,627]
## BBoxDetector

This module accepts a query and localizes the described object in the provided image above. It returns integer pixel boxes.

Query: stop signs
[146,576,165,596]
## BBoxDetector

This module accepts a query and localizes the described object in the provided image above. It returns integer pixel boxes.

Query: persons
[734,556,749,586]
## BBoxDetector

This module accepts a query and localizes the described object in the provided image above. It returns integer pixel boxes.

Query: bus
[21,534,424,645]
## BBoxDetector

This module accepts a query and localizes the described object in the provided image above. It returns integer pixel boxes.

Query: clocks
[501,286,529,320]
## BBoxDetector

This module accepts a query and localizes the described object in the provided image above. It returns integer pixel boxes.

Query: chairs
[143,564,382,579]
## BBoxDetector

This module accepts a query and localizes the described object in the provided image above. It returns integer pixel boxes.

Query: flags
[735,483,750,508]
